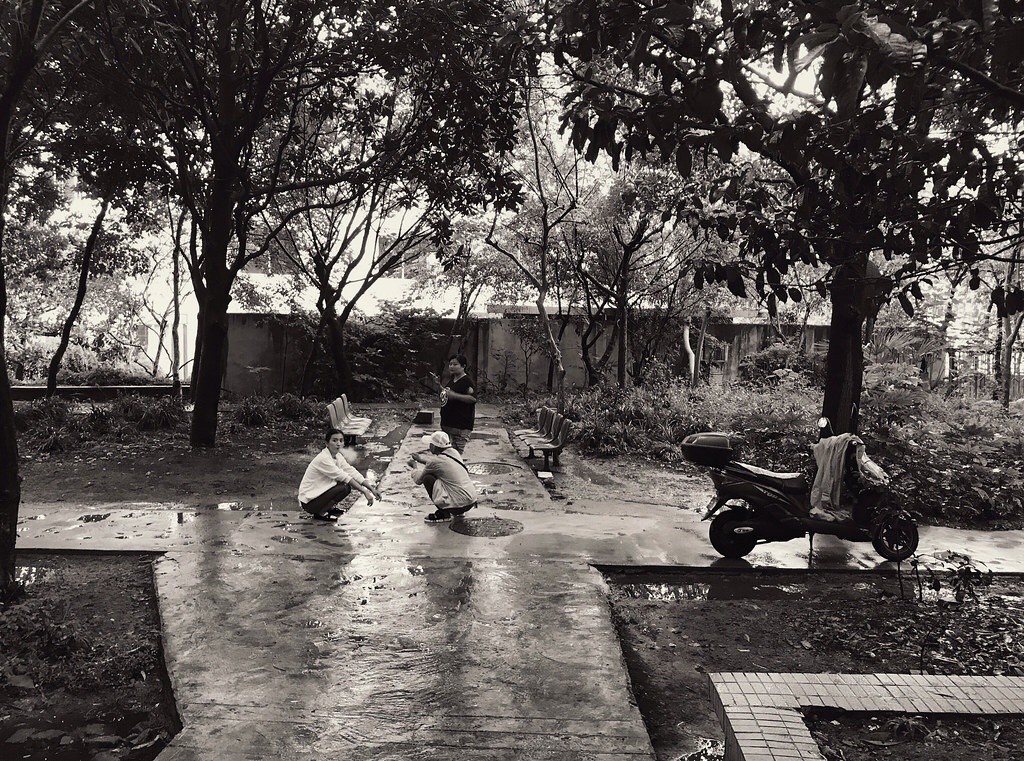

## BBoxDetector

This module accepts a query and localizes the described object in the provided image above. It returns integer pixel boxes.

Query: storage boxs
[417,411,434,425]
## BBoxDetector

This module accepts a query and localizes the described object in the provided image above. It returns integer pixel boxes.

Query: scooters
[680,403,919,562]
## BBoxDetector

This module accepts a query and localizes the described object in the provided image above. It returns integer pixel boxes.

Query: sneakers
[423,511,452,522]
[450,510,466,516]
[313,512,337,521]
[328,508,344,515]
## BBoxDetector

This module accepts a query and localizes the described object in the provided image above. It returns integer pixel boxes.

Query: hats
[421,431,451,448]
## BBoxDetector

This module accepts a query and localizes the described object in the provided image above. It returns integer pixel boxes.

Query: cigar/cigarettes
[430,372,438,378]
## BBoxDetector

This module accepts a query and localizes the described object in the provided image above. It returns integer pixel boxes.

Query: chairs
[514,405,572,473]
[326,394,373,448]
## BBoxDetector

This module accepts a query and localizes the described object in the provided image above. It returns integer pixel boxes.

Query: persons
[408,431,477,523]
[433,355,477,456]
[298,429,383,521]
